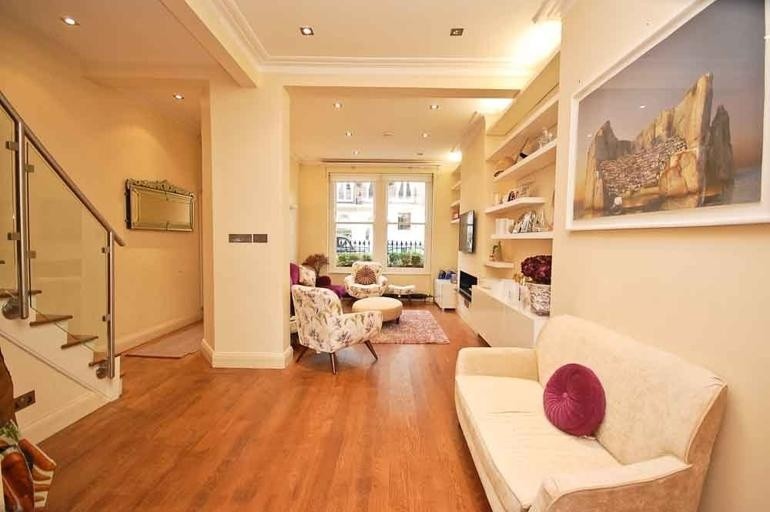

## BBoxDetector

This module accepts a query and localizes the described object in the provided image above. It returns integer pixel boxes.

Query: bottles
[338,181,411,199]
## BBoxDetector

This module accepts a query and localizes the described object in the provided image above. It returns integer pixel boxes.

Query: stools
[390,285,415,303]
[352,297,403,324]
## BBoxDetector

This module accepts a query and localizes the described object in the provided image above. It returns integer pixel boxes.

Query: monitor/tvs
[457,208,476,255]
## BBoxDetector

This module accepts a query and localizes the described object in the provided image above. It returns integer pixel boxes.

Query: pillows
[354,265,377,285]
[298,264,316,287]
[543,364,605,437]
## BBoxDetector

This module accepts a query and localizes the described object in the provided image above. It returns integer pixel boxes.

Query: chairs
[292,285,384,374]
[345,262,388,305]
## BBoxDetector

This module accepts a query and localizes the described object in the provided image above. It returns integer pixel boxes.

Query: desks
[434,279,455,312]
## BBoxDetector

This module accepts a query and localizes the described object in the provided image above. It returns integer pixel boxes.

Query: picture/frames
[565,0,770,232]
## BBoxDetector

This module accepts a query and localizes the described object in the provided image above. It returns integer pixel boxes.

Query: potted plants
[521,255,550,316]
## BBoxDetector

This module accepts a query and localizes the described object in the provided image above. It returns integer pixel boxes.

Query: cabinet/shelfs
[450,181,460,224]
[484,43,559,269]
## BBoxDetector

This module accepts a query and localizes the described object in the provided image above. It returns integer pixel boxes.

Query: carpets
[126,323,203,359]
[343,309,450,344]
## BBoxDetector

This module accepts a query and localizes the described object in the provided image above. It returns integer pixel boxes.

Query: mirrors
[124,175,195,233]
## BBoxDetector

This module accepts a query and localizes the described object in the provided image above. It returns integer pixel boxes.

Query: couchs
[290,262,347,351]
[454,314,727,512]
